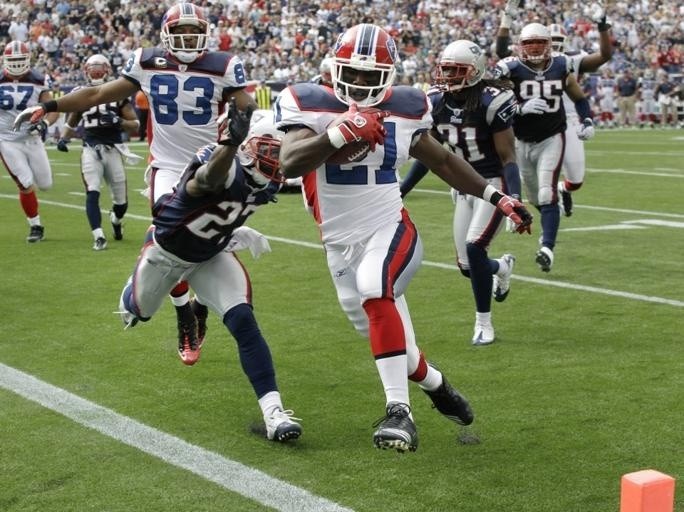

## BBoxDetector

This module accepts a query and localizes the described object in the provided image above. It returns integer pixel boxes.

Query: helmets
[159,2,210,62]
[515,22,551,64]
[330,23,398,110]
[83,54,112,86]
[236,109,287,189]
[548,24,568,54]
[2,41,30,74]
[435,39,486,92]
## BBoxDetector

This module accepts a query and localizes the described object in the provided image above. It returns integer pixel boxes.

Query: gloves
[576,117,594,142]
[505,1,525,18]
[485,187,534,236]
[99,110,123,128]
[55,135,71,154]
[327,98,390,150]
[10,103,47,132]
[216,95,256,145]
[516,96,549,115]
[590,5,610,31]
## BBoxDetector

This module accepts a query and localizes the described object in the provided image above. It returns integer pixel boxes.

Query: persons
[134,87,149,142]
[57,54,140,250]
[496,1,612,217]
[273,24,532,451]
[14,3,260,364]
[0,41,58,242]
[0,0,684,143]
[495,25,590,271]
[397,41,521,345]
[113,97,302,441]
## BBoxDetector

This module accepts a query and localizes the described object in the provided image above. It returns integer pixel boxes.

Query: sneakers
[468,320,495,346]
[173,301,209,365]
[24,225,46,244]
[118,275,140,331]
[422,360,481,428]
[92,238,106,252]
[110,215,126,241]
[370,406,419,453]
[492,254,514,302]
[534,249,553,272]
[260,405,302,442]
[557,181,574,217]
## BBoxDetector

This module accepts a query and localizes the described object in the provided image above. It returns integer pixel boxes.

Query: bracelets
[327,127,345,149]
[483,184,497,201]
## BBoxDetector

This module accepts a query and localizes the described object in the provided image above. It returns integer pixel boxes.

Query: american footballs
[320,108,383,164]
